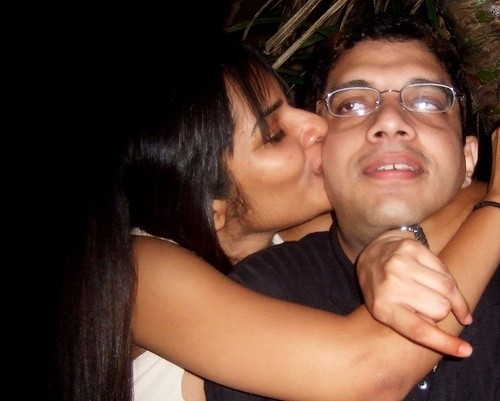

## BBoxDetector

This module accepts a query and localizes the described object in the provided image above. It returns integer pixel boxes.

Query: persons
[58,26,500,401]
[202,11,500,401]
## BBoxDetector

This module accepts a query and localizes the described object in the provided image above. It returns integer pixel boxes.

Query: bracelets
[472,201,500,211]
[391,223,431,253]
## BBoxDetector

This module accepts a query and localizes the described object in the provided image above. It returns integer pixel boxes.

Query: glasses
[315,83,467,128]
